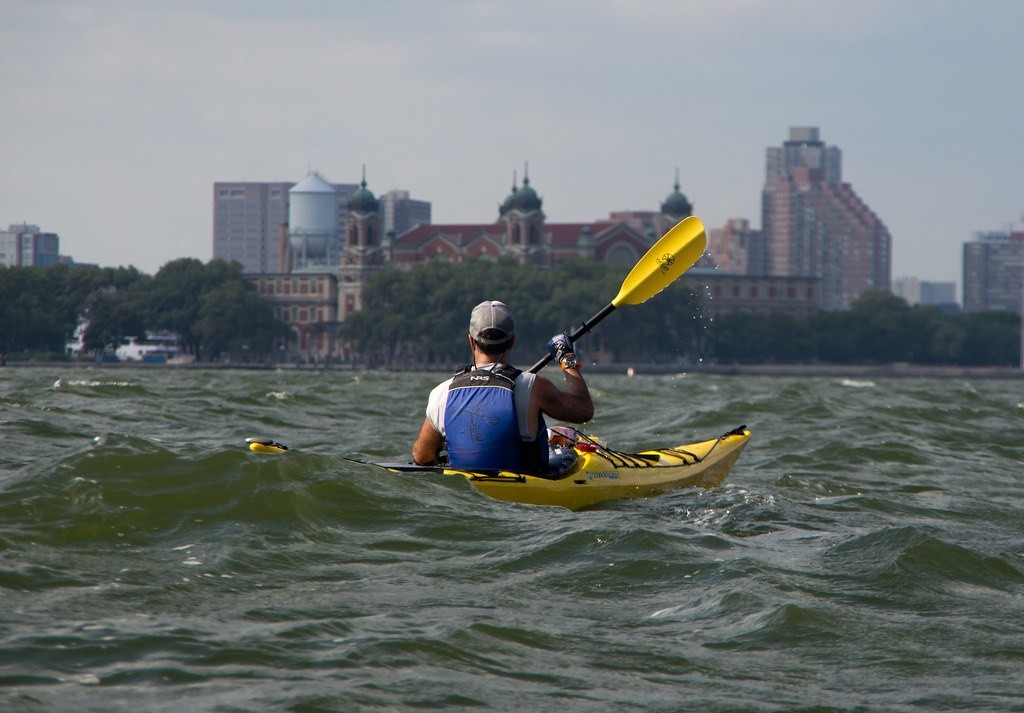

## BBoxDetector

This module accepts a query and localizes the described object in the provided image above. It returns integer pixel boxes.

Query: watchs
[562,356,583,370]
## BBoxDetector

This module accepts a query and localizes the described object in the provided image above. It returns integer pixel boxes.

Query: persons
[413,301,594,480]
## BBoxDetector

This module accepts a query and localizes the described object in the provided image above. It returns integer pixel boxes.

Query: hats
[469,300,515,345]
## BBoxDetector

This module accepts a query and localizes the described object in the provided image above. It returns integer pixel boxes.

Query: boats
[248,425,752,507]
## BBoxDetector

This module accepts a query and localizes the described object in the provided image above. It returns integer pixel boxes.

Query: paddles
[530,213,708,373]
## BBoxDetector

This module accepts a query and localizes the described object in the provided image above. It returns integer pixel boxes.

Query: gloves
[547,331,574,365]
